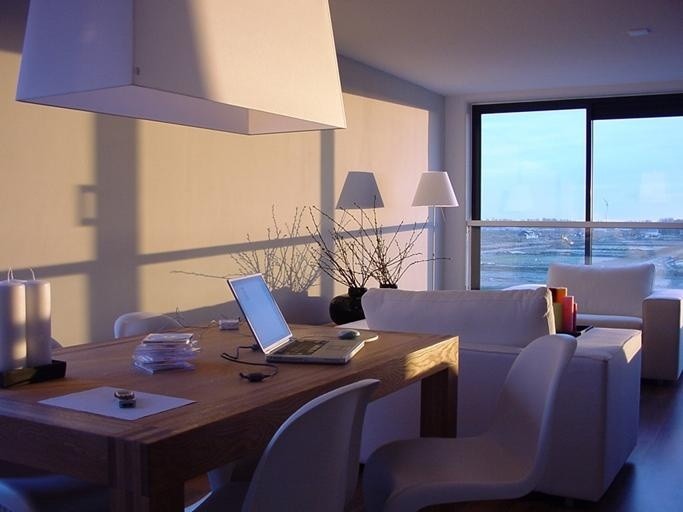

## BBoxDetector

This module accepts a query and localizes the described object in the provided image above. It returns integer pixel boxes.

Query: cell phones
[115,390,134,397]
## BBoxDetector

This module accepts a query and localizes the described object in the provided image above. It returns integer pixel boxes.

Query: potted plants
[303,196,454,324]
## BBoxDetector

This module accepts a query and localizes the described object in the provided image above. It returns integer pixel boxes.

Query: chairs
[113,310,185,340]
[190,377,383,512]
[359,334,579,511]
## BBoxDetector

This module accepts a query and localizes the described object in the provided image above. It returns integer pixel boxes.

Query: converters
[218,320,239,330]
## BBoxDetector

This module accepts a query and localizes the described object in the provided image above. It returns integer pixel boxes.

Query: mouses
[336,328,360,340]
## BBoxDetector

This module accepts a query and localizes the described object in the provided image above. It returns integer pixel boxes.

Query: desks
[0,323,459,512]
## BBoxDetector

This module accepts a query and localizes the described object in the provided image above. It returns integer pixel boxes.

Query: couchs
[502,262,682,382]
[334,285,644,502]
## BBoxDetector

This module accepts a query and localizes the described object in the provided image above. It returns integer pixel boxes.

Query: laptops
[227,273,365,365]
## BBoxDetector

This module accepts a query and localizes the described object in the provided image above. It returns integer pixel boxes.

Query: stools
[1,460,109,511]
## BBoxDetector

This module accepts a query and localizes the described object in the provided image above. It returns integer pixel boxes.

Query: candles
[25,266,51,368]
[0,264,25,371]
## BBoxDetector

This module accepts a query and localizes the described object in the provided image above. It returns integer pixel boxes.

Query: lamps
[410,171,460,287]
[14,0,350,136]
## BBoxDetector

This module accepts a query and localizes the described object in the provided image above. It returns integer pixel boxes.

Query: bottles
[0,279,53,371]
[548,287,578,334]
